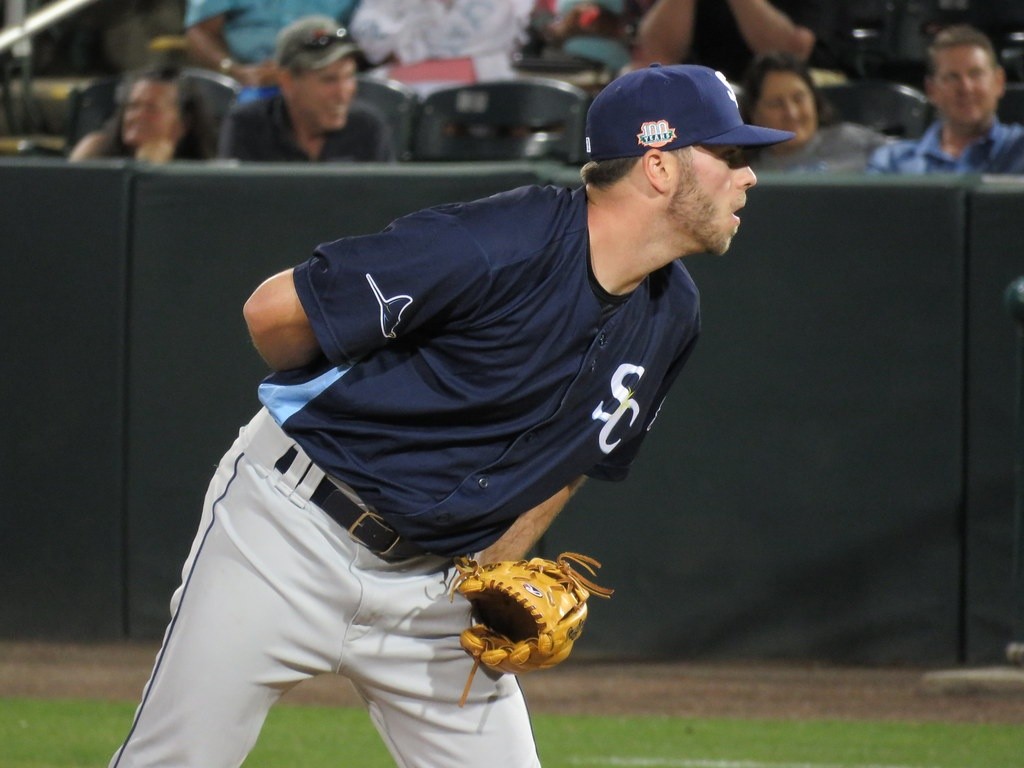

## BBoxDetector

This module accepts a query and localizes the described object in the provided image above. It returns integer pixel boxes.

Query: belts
[275,448,429,563]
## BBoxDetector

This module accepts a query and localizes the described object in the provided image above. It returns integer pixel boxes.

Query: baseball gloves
[457,557,590,676]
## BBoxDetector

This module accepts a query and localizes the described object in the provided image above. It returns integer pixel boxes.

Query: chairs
[77,68,1024,172]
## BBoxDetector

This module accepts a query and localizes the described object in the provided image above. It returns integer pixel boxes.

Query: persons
[68,70,211,164]
[628,0,816,78]
[868,26,1024,174]
[183,0,634,91]
[218,12,395,165]
[105,61,799,767]
[743,55,902,183]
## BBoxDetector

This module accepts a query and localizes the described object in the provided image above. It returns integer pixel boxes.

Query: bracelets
[219,58,235,75]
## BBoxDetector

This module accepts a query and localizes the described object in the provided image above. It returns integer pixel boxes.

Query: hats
[585,63,796,160]
[273,25,364,70]
